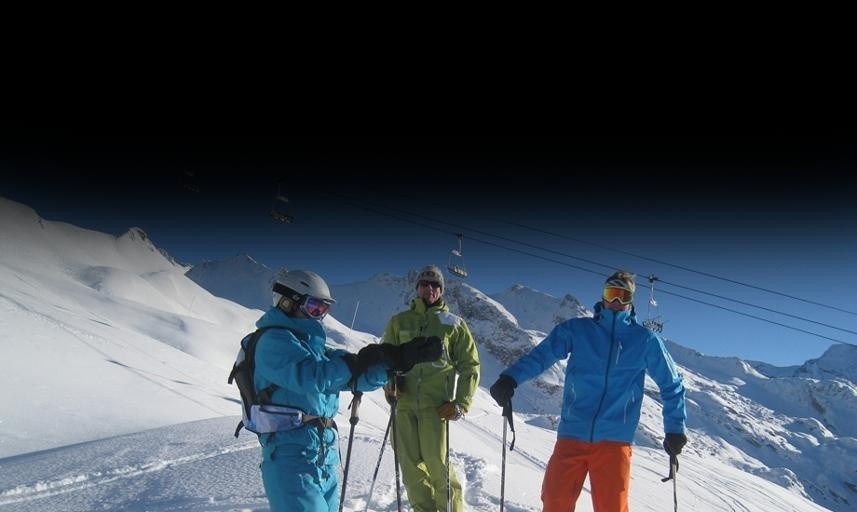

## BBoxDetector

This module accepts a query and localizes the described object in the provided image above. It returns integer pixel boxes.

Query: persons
[253,267,441,512]
[489,272,689,512]
[382,263,481,512]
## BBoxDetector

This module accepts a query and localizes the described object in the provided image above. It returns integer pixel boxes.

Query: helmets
[271,270,338,317]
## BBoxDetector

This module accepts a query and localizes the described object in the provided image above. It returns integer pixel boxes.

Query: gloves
[383,378,406,402]
[663,432,687,456]
[391,335,443,377]
[490,375,516,407]
[344,343,386,377]
[437,401,466,421]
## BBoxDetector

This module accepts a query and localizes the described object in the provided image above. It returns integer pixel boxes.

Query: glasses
[602,287,633,305]
[420,281,441,289]
[273,283,329,321]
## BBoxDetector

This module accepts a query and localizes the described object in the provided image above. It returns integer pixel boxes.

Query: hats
[415,265,445,293]
[605,271,635,293]
